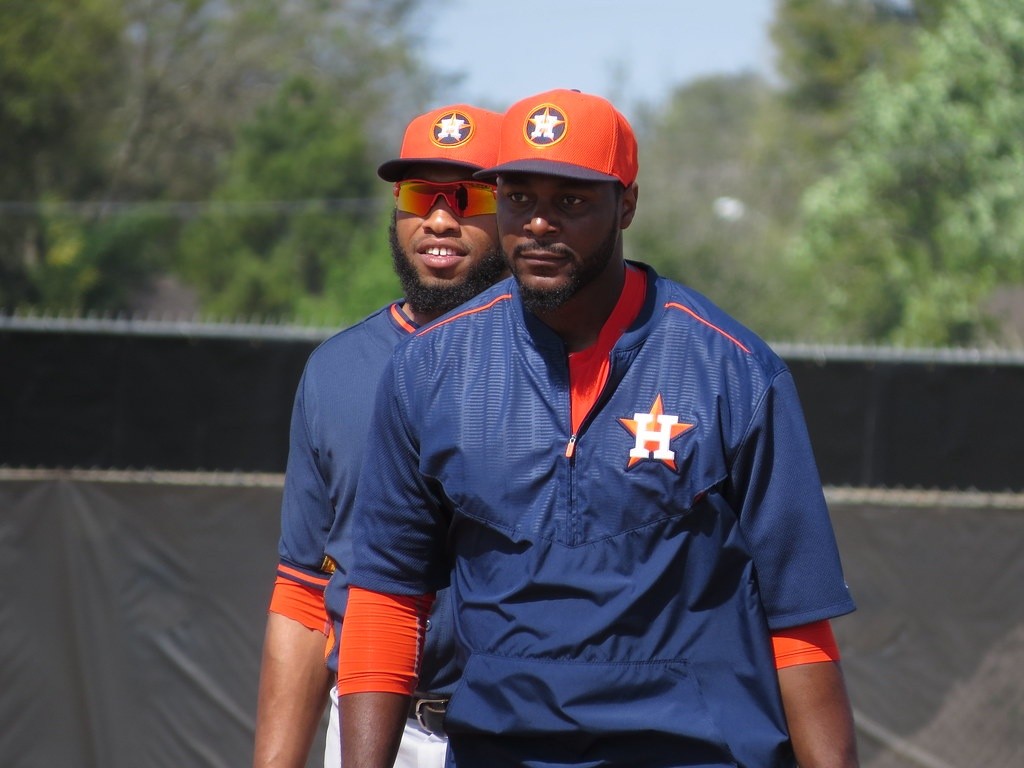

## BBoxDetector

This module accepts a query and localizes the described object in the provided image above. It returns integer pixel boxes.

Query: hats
[473,88,639,189]
[377,103,500,184]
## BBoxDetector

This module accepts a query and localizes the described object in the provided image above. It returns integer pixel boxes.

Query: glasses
[391,177,499,219]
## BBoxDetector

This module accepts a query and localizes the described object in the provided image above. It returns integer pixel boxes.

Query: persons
[336,88,860,768]
[252,103,502,768]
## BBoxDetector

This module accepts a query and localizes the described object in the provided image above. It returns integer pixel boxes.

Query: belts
[408,691,450,734]
